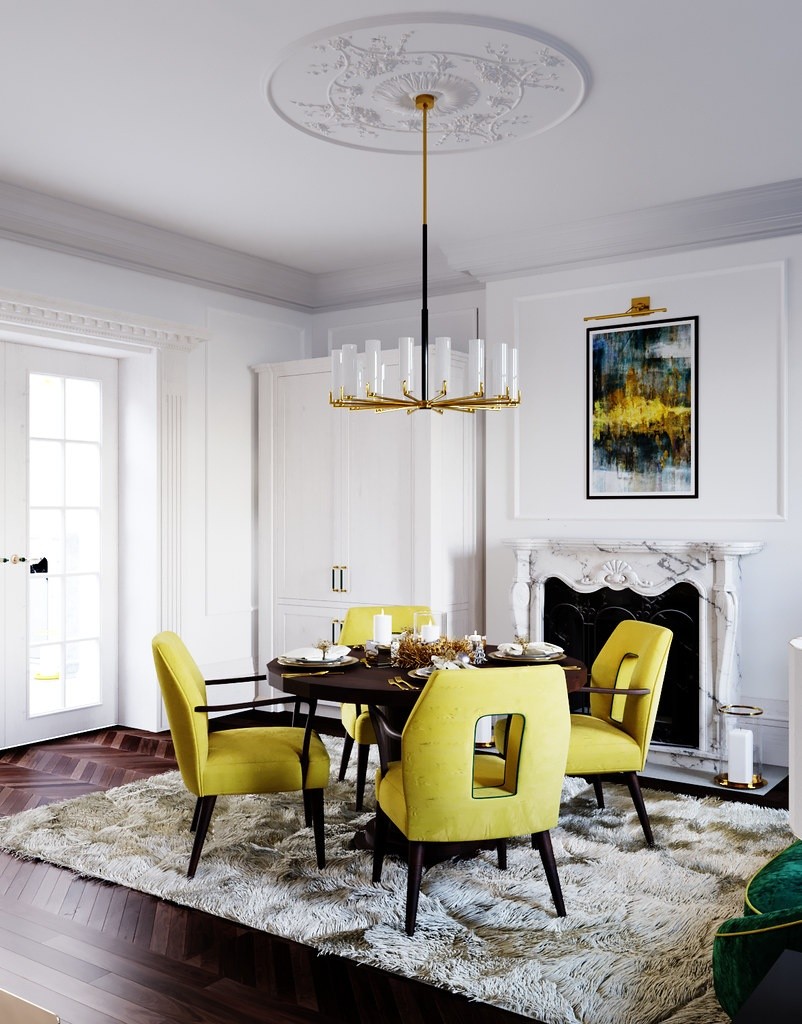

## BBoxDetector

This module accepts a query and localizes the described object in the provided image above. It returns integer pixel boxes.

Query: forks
[389,679,409,690]
[361,658,371,668]
[395,677,419,689]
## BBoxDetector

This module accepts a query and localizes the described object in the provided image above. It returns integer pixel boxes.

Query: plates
[278,645,359,667]
[489,643,567,661]
[408,665,478,679]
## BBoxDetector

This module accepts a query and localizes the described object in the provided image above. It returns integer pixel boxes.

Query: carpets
[0,735,802,1024]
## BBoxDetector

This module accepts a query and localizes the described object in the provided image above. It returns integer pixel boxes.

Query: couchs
[714,840,802,1024]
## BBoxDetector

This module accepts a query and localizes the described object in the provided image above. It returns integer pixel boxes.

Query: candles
[373,608,394,646]
[413,611,448,644]
[714,706,768,790]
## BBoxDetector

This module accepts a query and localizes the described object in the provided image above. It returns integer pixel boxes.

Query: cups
[414,611,447,648]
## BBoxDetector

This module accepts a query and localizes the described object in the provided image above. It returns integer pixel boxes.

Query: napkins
[280,645,351,661]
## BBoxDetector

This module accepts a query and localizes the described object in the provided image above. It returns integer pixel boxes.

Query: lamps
[330,93,525,415]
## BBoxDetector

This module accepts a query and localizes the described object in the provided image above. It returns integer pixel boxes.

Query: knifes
[281,671,328,677]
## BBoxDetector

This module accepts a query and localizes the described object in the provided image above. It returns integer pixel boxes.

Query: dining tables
[266,645,567,871]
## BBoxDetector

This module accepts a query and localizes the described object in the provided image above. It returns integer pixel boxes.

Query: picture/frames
[584,314,700,502]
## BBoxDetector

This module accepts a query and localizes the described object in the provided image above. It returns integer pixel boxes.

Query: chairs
[492,620,673,853]
[337,606,434,815]
[155,632,330,878]
[372,662,570,928]
